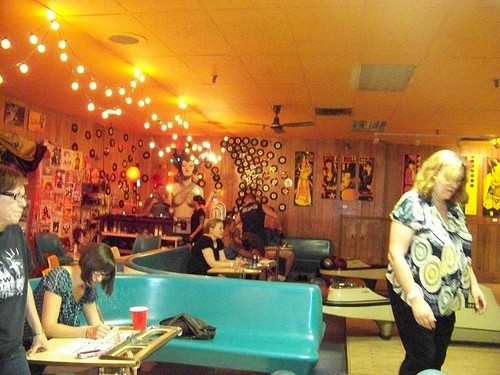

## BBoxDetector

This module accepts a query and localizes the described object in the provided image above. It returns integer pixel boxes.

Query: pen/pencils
[96,305,105,324]
[78,349,100,354]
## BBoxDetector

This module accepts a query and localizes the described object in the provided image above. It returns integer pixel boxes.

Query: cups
[130,306,148,330]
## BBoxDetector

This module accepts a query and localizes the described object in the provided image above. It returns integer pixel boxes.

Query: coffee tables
[313,257,397,339]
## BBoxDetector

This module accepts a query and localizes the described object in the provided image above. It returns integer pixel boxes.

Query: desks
[23,324,182,375]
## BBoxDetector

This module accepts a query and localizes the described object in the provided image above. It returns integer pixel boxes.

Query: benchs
[23,271,324,374]
[124,235,331,280]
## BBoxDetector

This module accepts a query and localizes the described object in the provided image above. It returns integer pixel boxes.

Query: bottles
[252,253,257,269]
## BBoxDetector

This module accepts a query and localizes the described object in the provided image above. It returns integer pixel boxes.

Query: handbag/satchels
[320,255,347,270]
[159,313,216,341]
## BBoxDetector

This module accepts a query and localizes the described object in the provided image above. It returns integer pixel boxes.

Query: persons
[187,194,207,245]
[187,219,243,278]
[52,148,80,189]
[0,164,49,375]
[483,157,500,216]
[222,188,295,281]
[20,242,118,349]
[384,149,486,375]
[142,183,172,219]
[322,159,416,202]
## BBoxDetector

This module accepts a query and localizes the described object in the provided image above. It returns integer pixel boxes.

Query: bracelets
[32,329,45,335]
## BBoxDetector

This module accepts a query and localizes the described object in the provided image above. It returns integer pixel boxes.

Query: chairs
[34,232,73,276]
[129,234,162,256]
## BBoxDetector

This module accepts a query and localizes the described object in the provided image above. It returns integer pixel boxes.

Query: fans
[235,104,316,136]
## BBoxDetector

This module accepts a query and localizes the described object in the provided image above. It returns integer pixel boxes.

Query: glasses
[94,268,110,280]
[440,172,463,185]
[0,191,28,201]
